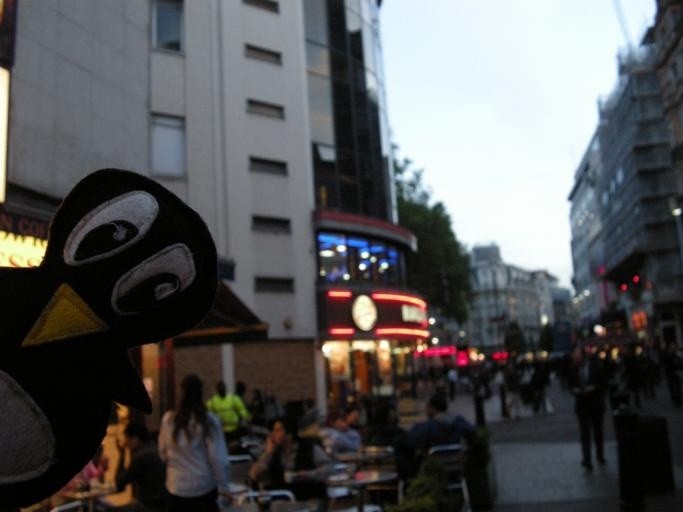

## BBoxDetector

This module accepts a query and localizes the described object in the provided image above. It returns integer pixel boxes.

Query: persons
[468,346,681,472]
[74,371,493,512]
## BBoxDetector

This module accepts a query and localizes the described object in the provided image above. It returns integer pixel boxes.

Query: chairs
[51,423,473,512]
